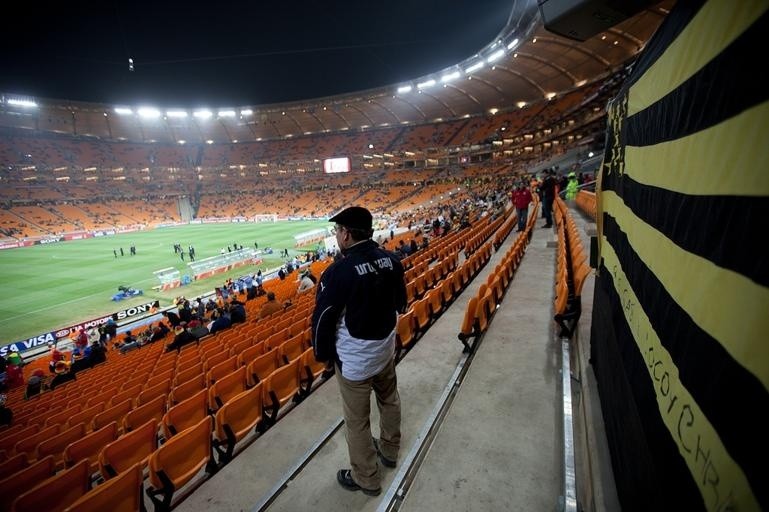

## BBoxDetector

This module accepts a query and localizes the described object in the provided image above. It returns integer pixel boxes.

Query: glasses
[330,225,343,235]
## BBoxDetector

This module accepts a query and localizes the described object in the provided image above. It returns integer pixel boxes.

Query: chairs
[0,300,429,512]
[199,182,598,331]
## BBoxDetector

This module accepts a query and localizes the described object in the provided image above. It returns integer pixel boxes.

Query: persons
[0,300,170,432]
[113,245,136,259]
[0,89,608,237]
[169,237,430,351]
[307,206,411,497]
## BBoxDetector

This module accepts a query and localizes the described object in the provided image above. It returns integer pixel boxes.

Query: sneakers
[372,437,397,468]
[337,469,381,496]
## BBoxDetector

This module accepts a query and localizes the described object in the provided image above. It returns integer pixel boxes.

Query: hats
[328,207,373,229]
